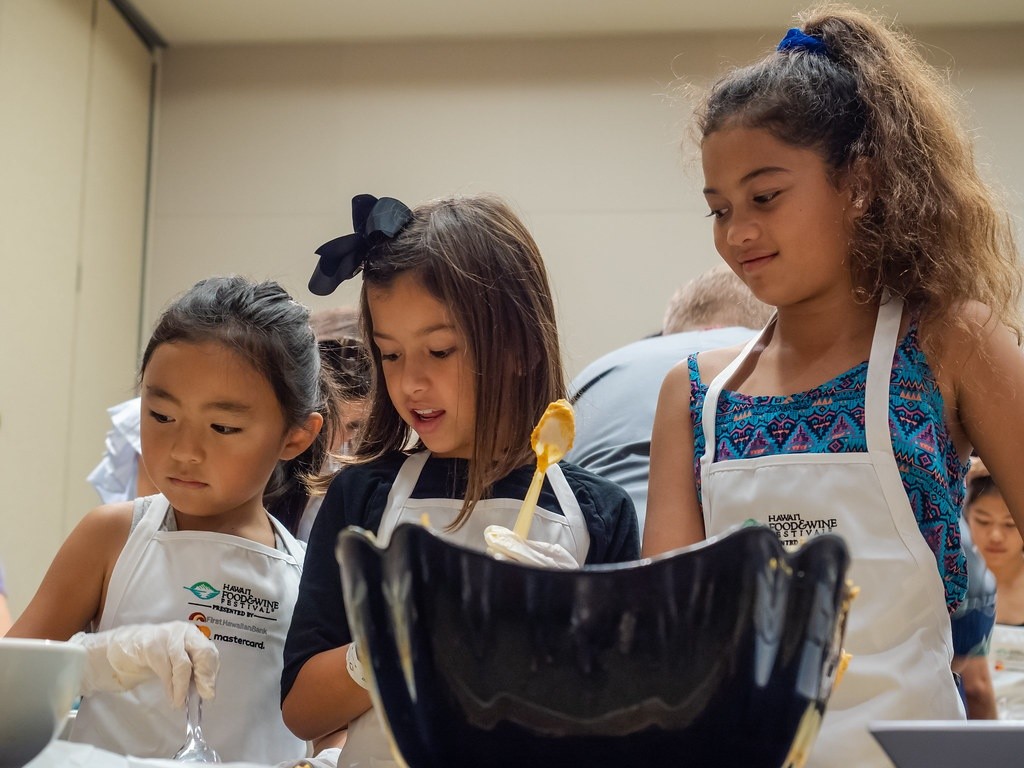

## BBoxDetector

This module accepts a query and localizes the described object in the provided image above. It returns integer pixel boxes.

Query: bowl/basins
[867,720,1024,768]
[0,635,90,768]
[333,520,856,768]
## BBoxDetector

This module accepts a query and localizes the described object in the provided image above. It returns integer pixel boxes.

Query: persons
[558,260,778,551]
[637,0,1022,768]
[278,199,643,768]
[4,274,376,768]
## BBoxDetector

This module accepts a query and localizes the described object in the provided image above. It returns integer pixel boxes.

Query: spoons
[513,399,582,539]
[171,677,223,768]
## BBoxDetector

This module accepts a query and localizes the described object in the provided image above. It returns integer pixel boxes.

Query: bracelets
[346,640,370,692]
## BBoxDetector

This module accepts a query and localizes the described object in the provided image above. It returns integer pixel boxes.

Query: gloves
[277,746,342,768]
[484,524,581,571]
[68,621,220,712]
